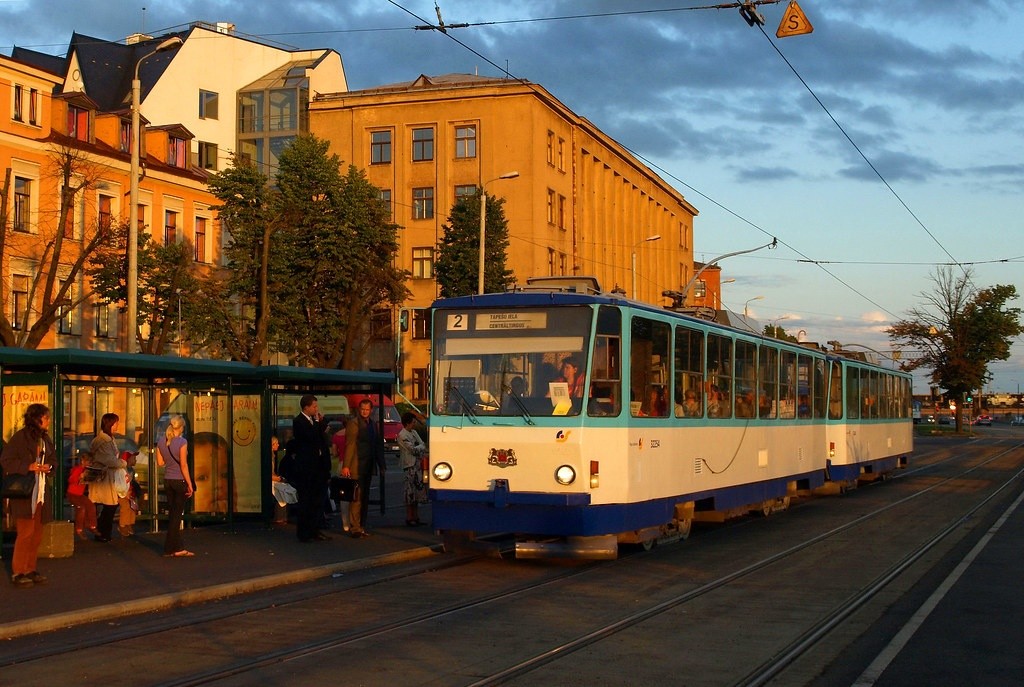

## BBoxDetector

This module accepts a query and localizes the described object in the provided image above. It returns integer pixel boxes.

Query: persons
[637,382,813,418]
[194,432,238,512]
[332,399,386,539]
[396,412,428,528]
[65,412,141,542]
[0,403,59,588]
[546,356,592,398]
[272,395,334,542]
[155,415,195,558]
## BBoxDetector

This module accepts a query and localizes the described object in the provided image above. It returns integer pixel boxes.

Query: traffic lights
[967,395,972,403]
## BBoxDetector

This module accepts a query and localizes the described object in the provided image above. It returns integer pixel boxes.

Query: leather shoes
[304,533,333,544]
[360,530,372,536]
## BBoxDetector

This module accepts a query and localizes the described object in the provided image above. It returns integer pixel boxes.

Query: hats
[122,452,139,461]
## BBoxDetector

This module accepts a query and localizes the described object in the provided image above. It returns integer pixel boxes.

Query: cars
[1008,415,1024,427]
[925,406,992,427]
[52,433,137,476]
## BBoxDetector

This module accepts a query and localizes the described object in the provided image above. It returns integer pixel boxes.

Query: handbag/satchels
[1,472,36,499]
[115,468,130,499]
[182,477,196,491]
[328,474,358,502]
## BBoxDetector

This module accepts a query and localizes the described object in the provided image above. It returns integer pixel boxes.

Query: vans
[342,393,404,457]
[129,394,348,492]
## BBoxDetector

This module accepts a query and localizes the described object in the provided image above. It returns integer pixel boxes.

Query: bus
[427,289,915,563]
[911,400,922,424]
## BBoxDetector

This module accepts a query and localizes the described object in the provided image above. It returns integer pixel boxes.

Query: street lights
[1011,379,1019,422]
[631,234,661,300]
[774,313,802,338]
[125,36,184,394]
[478,172,520,295]
[743,295,764,383]
[713,278,735,309]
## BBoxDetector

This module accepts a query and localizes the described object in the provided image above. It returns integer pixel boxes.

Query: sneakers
[117,525,134,536]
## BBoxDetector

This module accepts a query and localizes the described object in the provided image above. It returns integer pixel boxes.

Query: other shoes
[172,550,194,557]
[76,529,87,540]
[12,571,47,586]
[88,527,101,537]
